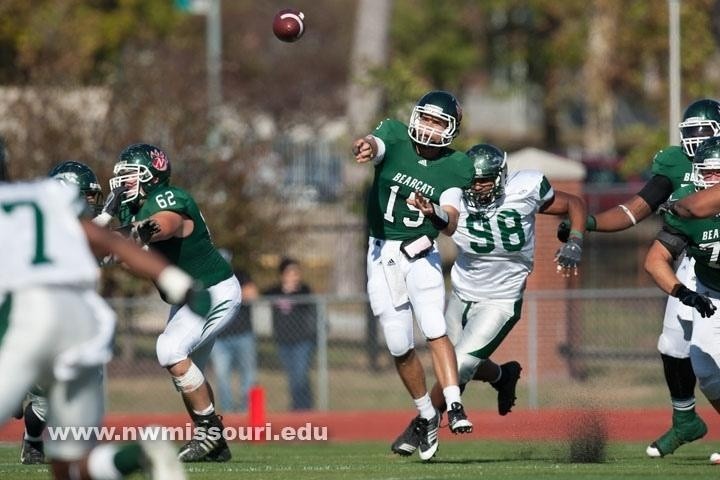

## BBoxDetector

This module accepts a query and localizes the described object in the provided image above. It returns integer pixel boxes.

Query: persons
[259,257,321,413]
[350,89,477,466]
[555,97,719,458]
[644,134,719,465]
[98,143,244,464]
[653,181,720,220]
[0,141,209,480]
[8,159,159,464]
[204,242,259,412]
[388,145,589,456]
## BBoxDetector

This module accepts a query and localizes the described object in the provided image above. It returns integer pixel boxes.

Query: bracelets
[429,202,449,230]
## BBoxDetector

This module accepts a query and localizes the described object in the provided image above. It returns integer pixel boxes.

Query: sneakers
[489,360,522,416]
[135,422,190,480]
[446,401,474,436]
[645,405,708,460]
[709,451,720,464]
[19,431,46,465]
[176,410,233,463]
[390,407,444,464]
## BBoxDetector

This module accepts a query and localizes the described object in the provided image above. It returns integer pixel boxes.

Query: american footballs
[271,8,307,43]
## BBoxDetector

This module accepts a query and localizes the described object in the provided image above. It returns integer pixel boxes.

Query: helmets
[464,143,509,200]
[681,98,720,128]
[415,89,463,141]
[48,159,104,195]
[115,141,172,195]
[692,135,720,169]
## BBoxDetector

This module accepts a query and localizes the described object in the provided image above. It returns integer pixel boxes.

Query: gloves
[154,262,196,308]
[127,217,163,248]
[671,283,717,319]
[100,185,138,218]
[553,214,597,279]
